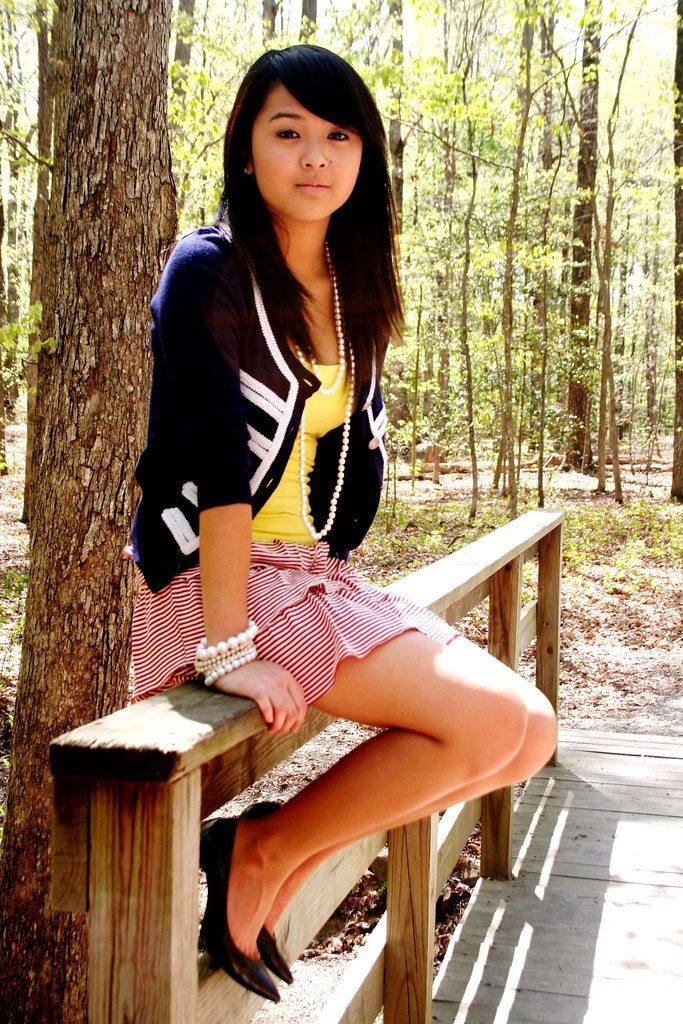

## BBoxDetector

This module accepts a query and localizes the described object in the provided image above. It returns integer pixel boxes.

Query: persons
[127,42,560,1006]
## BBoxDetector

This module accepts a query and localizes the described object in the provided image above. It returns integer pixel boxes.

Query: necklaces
[287,235,357,543]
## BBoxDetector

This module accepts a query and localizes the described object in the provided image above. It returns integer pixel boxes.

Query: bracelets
[193,620,260,687]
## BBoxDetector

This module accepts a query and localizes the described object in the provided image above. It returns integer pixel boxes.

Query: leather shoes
[196,817,281,1005]
[230,800,295,984]
